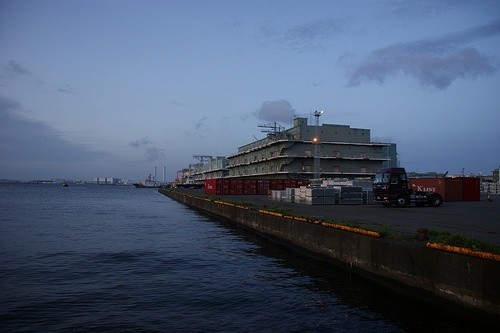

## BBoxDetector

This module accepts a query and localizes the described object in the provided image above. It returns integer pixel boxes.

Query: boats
[133,174,164,189]
[61,182,68,187]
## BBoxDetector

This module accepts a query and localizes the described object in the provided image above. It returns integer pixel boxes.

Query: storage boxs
[410,176,464,201]
[462,177,480,201]
[204,178,375,205]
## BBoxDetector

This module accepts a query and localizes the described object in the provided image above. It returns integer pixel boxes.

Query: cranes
[258,121,283,134]
[189,154,212,164]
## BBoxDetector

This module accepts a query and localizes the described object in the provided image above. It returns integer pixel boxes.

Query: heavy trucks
[371,166,444,208]
